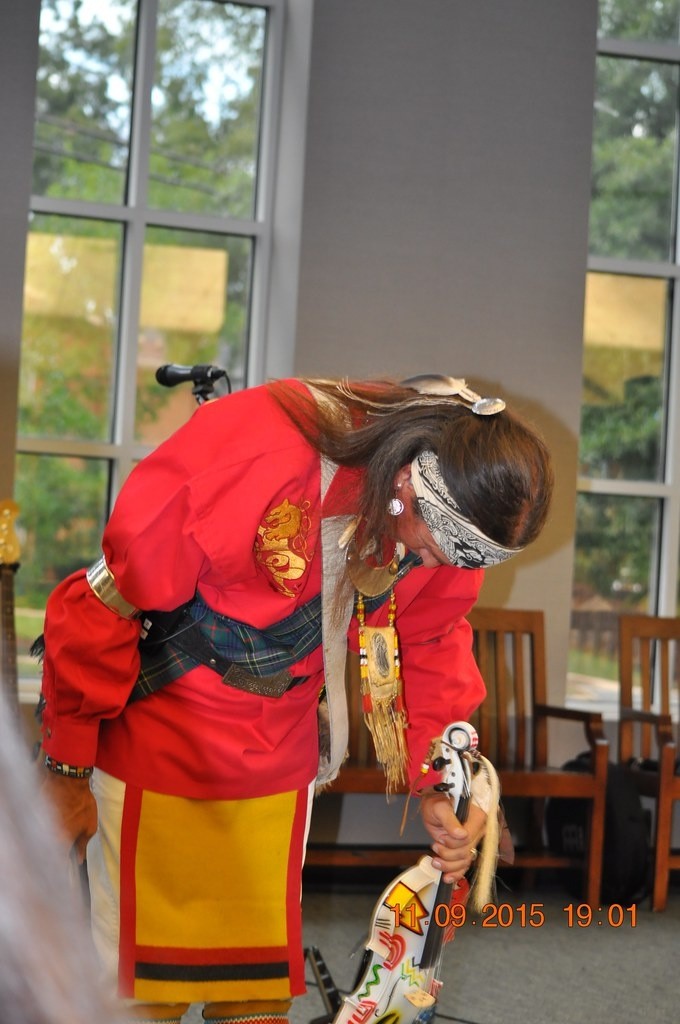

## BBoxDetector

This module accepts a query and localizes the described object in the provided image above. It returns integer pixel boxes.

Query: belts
[166,615,310,697]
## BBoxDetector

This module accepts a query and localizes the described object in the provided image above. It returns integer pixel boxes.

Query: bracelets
[45,754,94,779]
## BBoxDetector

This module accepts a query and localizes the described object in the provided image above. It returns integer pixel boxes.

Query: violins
[331,721,481,1024]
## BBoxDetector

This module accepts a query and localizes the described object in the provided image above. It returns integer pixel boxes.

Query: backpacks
[546,750,652,908]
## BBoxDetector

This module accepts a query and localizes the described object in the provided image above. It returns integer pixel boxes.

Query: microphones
[155,365,226,388]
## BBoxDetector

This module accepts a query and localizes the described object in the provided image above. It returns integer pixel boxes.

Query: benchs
[307,607,680,913]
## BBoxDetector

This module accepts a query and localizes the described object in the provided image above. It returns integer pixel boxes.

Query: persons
[31,375,554,1023]
